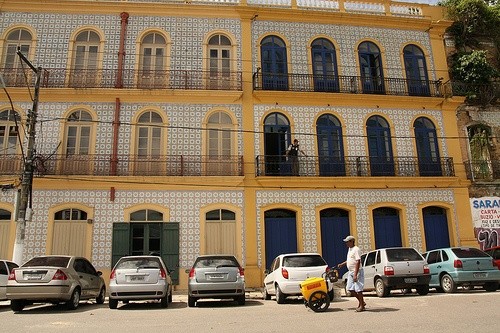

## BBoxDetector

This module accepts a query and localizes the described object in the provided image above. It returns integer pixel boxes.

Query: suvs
[342,246,431,298]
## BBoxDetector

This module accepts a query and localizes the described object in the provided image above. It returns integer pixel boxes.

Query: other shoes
[356,306,365,312]
[356,302,366,309]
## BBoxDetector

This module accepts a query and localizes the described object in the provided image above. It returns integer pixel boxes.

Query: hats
[343,236,356,243]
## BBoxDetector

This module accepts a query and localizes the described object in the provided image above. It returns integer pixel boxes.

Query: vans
[263,252,336,305]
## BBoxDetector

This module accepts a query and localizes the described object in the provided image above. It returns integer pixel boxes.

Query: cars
[0,254,108,312]
[185,254,247,307]
[107,256,176,309]
[422,246,500,294]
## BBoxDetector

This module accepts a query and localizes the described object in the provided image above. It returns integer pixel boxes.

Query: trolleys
[298,264,339,312]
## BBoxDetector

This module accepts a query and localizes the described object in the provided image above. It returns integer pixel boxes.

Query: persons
[338,236,366,312]
[285,139,307,176]
